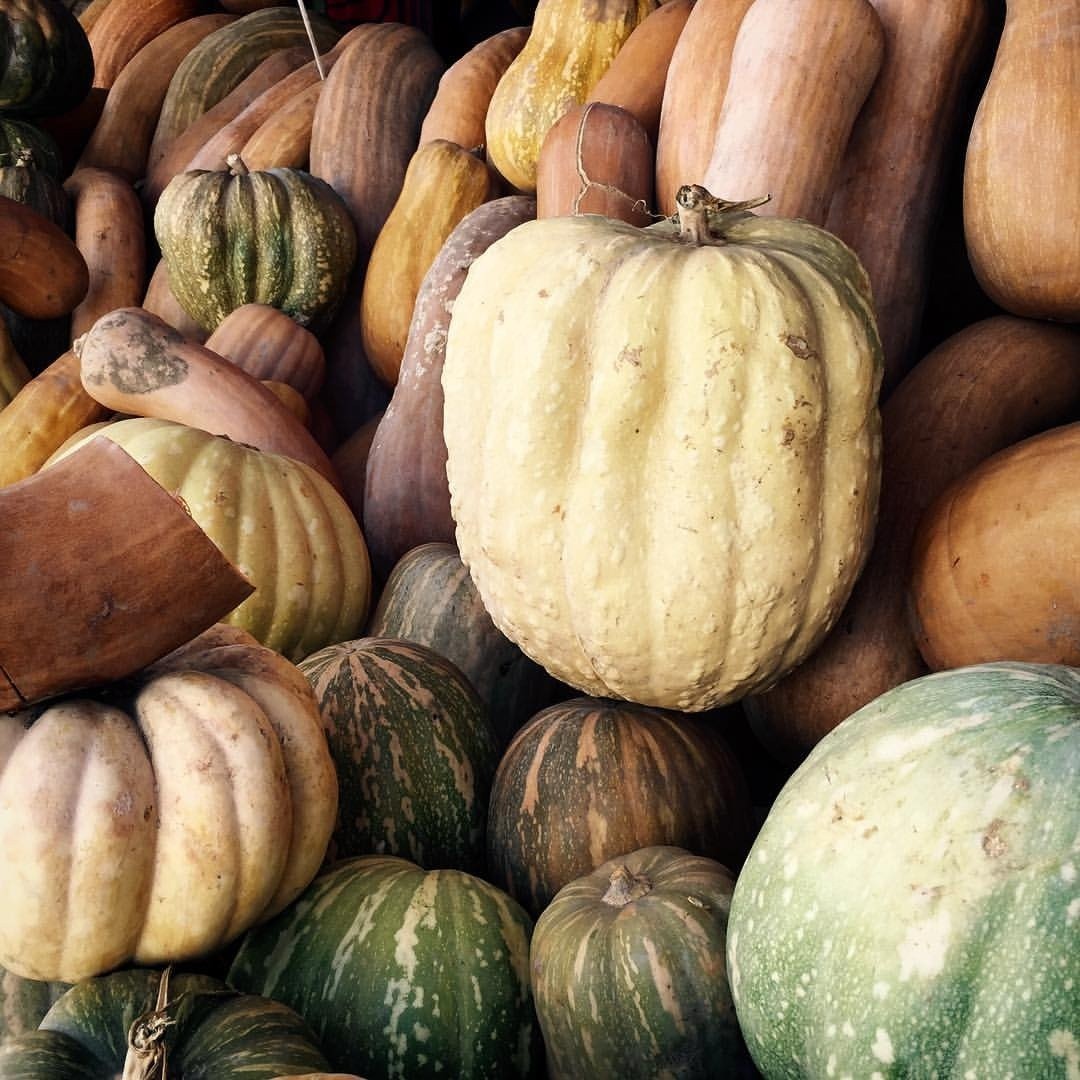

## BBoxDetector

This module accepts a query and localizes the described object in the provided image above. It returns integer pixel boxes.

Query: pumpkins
[0,0,1080,1079]
